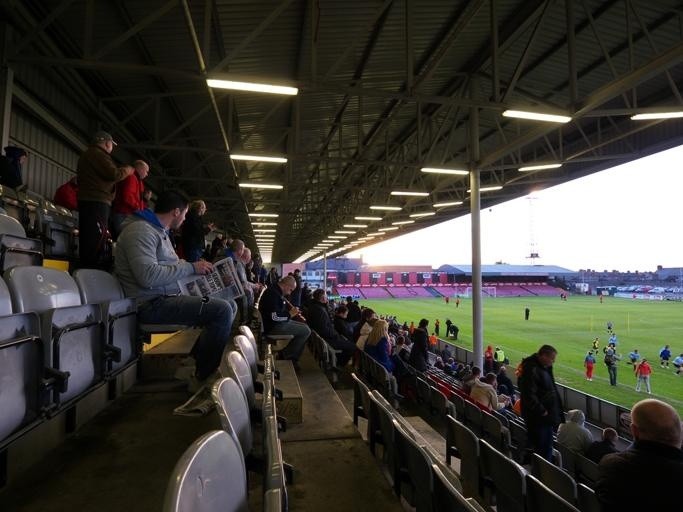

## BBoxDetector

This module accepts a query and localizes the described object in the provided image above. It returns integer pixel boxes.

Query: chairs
[3,265,103,414]
[477,440,528,511]
[72,268,138,377]
[577,482,595,508]
[429,464,483,511]
[556,443,581,471]
[552,448,563,472]
[163,430,289,511]
[525,473,582,512]
[0,277,47,446]
[212,378,294,481]
[445,414,481,482]
[578,453,599,480]
[1,187,137,286]
[137,185,682,441]
[531,452,577,508]
[390,419,464,511]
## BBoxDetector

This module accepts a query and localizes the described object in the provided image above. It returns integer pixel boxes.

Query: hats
[4,147,27,155]
[93,131,117,146]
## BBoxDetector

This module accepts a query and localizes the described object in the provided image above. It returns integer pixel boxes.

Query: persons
[54,133,311,397]
[583,331,622,388]
[595,398,683,512]
[599,294,602,304]
[660,345,671,369]
[628,349,640,370]
[525,306,530,320]
[673,354,683,375]
[606,320,613,334]
[310,288,459,376]
[455,343,620,484]
[0,144,35,239]
[560,292,567,301]
[635,359,651,394]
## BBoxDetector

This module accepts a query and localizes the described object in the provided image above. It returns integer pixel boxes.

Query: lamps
[206,71,300,97]
[629,112,683,121]
